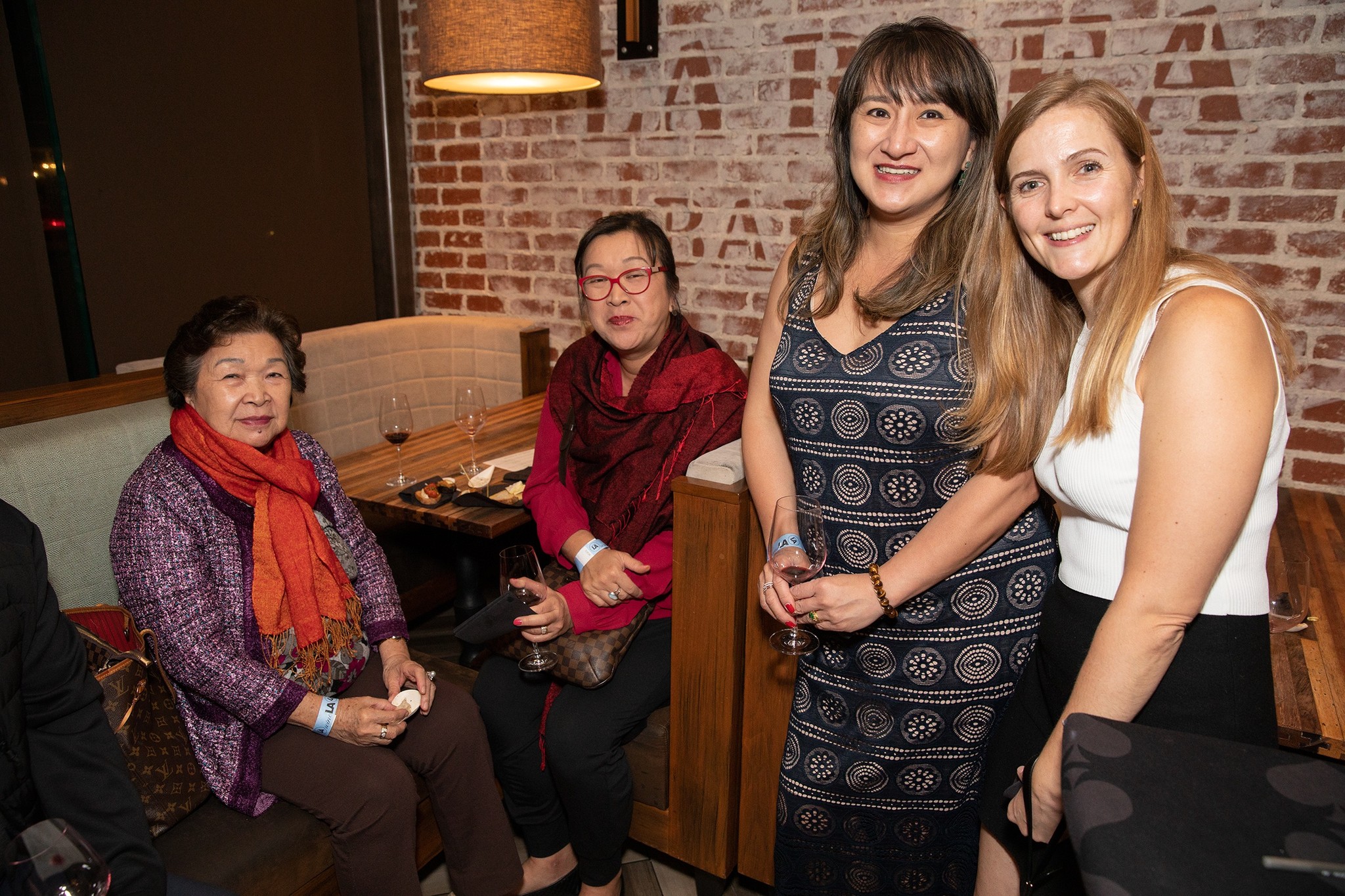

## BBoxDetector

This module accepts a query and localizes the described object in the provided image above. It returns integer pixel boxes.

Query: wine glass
[377,392,417,488]
[1265,542,1311,635]
[1,817,111,896]
[762,497,827,656]
[451,384,490,474]
[498,544,558,672]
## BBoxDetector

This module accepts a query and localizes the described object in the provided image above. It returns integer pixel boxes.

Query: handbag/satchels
[60,602,212,839]
[487,560,659,690]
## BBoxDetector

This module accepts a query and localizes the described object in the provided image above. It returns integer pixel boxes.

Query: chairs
[736,500,798,890]
[283,314,549,634]
[621,440,748,891]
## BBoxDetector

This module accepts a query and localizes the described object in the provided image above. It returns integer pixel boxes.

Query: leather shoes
[577,874,625,896]
[521,860,582,896]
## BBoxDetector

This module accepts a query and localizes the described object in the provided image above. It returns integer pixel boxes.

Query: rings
[540,625,548,635]
[809,610,825,623]
[424,671,436,680]
[380,724,388,739]
[763,582,774,595]
[608,587,621,600]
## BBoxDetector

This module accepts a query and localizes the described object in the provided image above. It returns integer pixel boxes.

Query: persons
[109,295,525,896]
[0,502,166,896]
[743,16,1084,896]
[944,74,1290,896]
[470,211,750,895]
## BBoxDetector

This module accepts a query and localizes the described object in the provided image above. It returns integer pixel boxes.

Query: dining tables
[330,387,549,625]
[1266,484,1345,764]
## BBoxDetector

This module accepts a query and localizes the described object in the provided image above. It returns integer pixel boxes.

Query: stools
[148,648,484,896]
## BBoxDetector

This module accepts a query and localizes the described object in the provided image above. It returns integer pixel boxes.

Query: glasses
[578,266,669,301]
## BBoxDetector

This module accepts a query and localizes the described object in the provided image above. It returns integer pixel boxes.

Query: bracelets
[312,695,339,737]
[573,538,611,574]
[766,533,807,562]
[869,563,898,618]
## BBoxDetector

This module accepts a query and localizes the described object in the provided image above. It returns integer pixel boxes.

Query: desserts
[414,482,441,505]
[437,480,457,493]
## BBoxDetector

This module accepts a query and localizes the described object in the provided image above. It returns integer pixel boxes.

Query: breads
[489,480,526,505]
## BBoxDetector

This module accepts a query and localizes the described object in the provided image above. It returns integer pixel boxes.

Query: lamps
[412,0,605,99]
